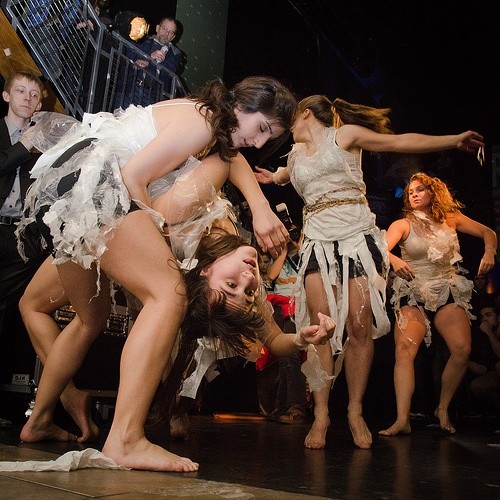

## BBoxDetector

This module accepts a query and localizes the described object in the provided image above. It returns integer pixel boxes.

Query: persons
[1,71,43,420]
[19,147,385,444]
[74,5,102,65]
[256,249,273,278]
[377,173,500,437]
[268,222,303,300]
[123,17,179,105]
[253,96,482,448]
[20,78,296,472]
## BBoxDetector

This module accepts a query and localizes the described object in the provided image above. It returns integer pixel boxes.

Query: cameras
[276,203,301,247]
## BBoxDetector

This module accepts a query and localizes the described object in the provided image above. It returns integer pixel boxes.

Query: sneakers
[278,406,308,423]
